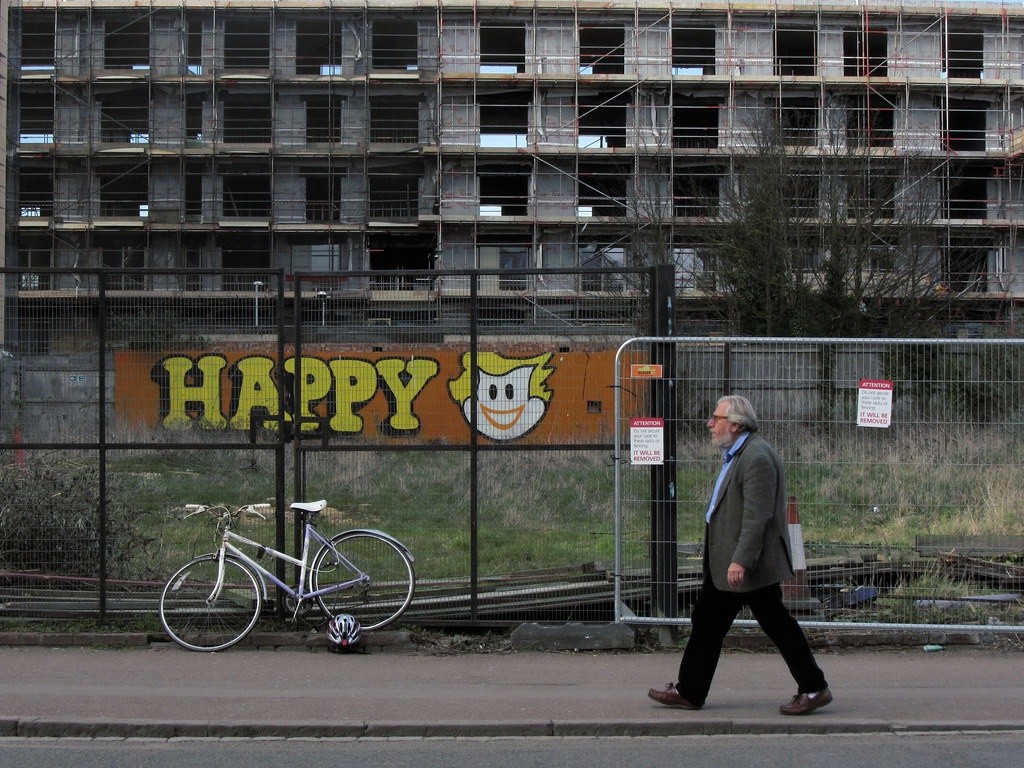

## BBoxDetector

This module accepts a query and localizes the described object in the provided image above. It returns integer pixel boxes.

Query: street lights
[316,289,328,326]
[253,280,265,326]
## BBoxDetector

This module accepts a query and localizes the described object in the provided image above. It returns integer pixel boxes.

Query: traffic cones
[782,495,822,610]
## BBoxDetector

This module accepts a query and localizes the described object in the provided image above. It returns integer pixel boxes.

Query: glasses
[712,415,728,423]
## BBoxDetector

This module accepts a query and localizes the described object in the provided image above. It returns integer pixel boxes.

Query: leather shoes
[780,687,833,715]
[648,683,702,710]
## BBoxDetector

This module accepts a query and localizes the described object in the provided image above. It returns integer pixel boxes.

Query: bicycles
[160,499,417,652]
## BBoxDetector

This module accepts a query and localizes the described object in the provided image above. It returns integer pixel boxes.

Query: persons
[648,395,833,716]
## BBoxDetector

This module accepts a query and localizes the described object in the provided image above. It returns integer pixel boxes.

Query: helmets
[327,613,361,654]
